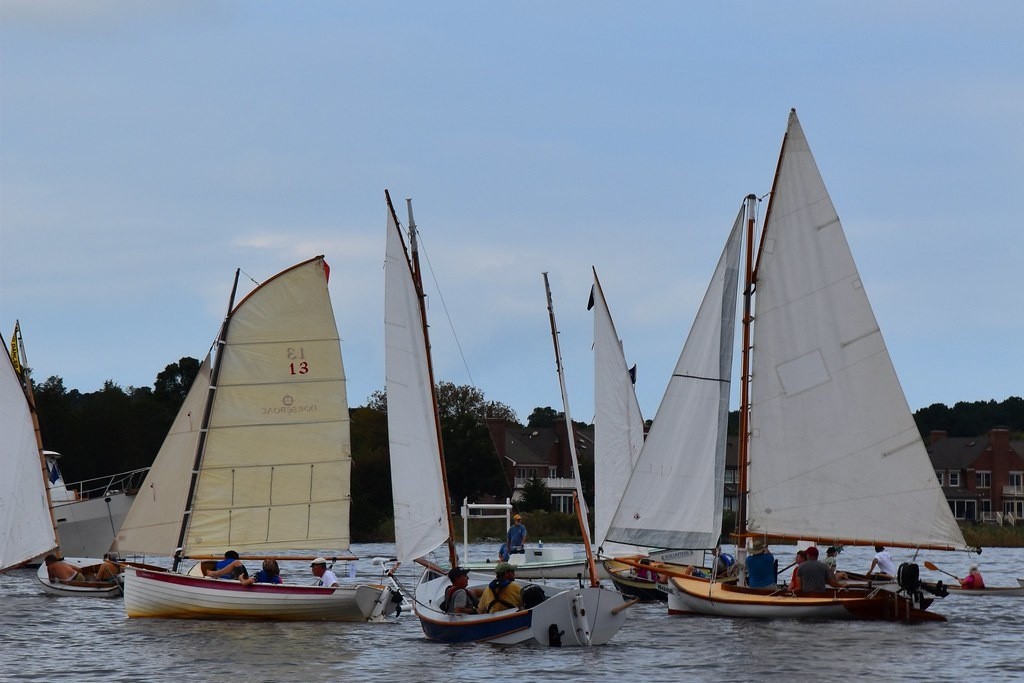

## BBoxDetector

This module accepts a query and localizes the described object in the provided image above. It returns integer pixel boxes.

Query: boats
[24,450,152,573]
[824,568,918,590]
[919,577,1024,598]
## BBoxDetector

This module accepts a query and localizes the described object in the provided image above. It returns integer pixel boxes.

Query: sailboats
[383,187,641,646]
[585,265,750,603]
[1,318,126,598]
[103,254,404,624]
[590,106,982,622]
[455,262,707,602]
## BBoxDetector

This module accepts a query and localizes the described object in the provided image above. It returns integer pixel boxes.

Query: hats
[310,558,326,567]
[495,562,517,573]
[747,541,764,554]
[803,547,818,556]
[448,567,470,580]
[514,515,521,519]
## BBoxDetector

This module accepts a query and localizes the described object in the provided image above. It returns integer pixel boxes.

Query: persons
[479,562,522,613]
[94,554,120,581]
[787,550,807,591]
[507,514,527,554]
[217,550,240,578]
[310,557,339,587]
[498,543,509,562]
[823,547,848,580]
[745,541,779,590]
[797,547,848,594]
[638,557,668,581]
[711,546,735,578]
[955,566,985,590]
[866,546,898,577]
[206,559,283,584]
[444,567,480,614]
[45,554,85,583]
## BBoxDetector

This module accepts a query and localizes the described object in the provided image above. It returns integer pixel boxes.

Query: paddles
[924,562,960,580]
